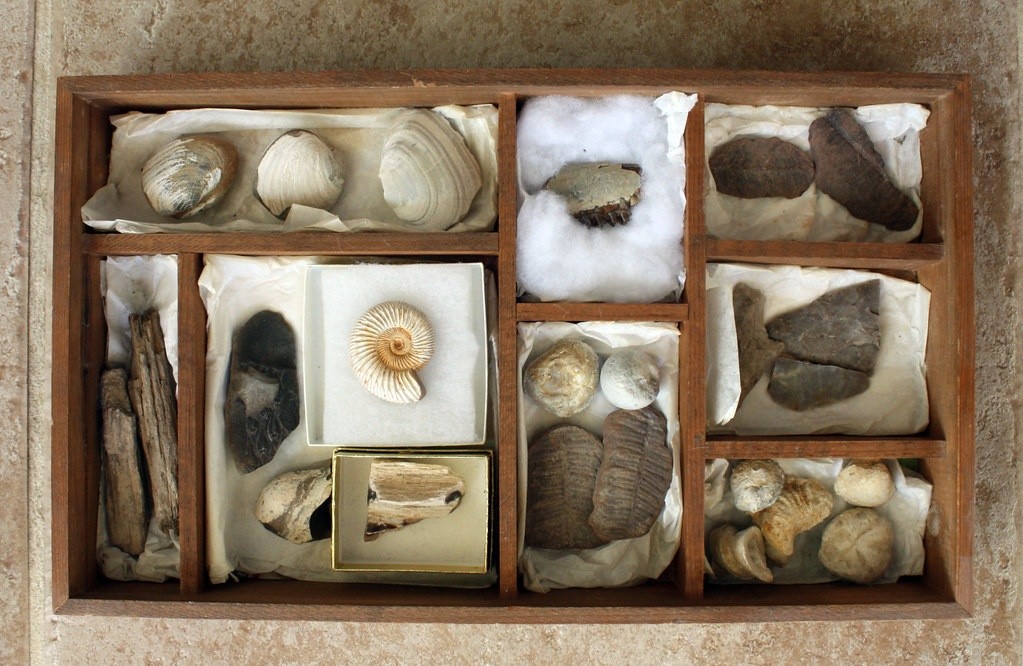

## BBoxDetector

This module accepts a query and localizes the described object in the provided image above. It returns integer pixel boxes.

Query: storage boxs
[51,72,975,623]
